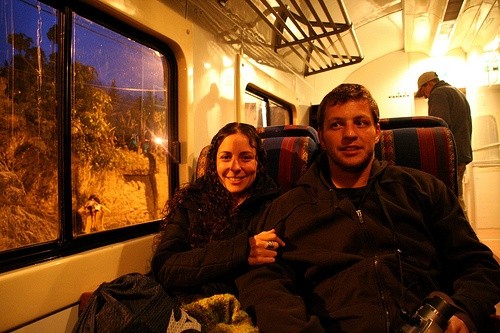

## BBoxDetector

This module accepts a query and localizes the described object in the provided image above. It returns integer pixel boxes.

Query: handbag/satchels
[71,273,203,333]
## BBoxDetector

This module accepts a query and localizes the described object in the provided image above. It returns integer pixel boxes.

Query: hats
[414,71,439,97]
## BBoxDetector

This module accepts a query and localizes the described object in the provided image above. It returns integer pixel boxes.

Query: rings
[267,241,274,250]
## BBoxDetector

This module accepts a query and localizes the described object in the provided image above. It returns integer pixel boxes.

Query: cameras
[400,295,453,333]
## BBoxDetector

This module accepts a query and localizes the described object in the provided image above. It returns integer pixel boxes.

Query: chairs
[193,116,458,202]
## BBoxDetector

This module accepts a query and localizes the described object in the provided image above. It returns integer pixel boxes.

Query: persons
[239,84,500,333]
[152,123,285,293]
[415,72,473,224]
[77,194,105,234]
